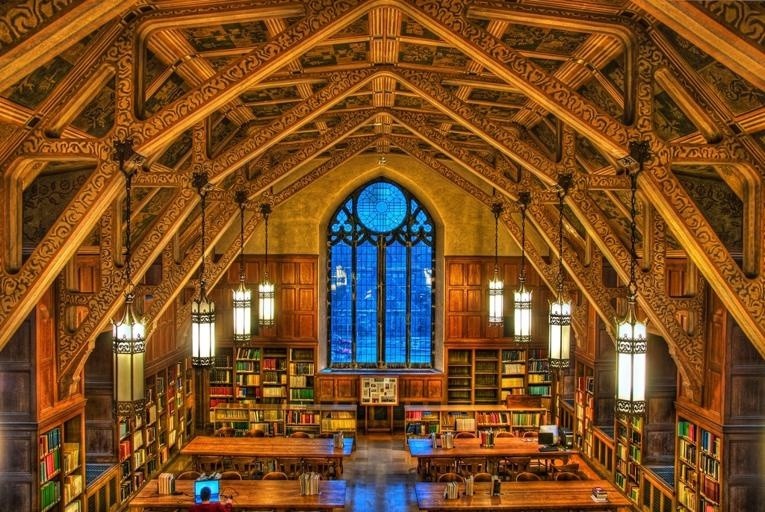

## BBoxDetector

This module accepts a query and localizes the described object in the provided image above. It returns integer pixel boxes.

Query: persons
[191,486,232,511]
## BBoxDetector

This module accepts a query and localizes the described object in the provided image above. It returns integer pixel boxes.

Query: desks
[130,478,347,512]
[414,480,633,512]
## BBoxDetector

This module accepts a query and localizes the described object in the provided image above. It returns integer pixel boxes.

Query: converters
[172,491,183,495]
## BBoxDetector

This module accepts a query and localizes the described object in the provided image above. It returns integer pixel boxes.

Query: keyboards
[539,447,558,452]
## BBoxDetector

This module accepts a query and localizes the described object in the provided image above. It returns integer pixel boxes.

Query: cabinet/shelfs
[574,357,595,464]
[115,418,150,506]
[673,408,723,512]
[205,348,233,430]
[234,346,262,405]
[613,412,642,508]
[404,405,547,449]
[557,400,575,446]
[288,344,316,403]
[37,402,86,512]
[639,466,674,512]
[214,403,357,450]
[316,374,358,402]
[399,375,442,402]
[261,344,288,404]
[591,425,614,480]
[146,351,196,479]
[445,343,551,410]
[85,466,120,512]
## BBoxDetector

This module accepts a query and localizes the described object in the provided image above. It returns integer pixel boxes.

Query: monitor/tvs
[539,424,558,446]
[538,433,553,448]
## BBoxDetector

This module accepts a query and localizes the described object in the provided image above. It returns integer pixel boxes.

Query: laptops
[194,478,220,504]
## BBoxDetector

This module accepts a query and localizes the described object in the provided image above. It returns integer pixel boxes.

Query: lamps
[488,203,505,328]
[613,141,651,425]
[192,170,217,370]
[512,191,534,348]
[548,174,576,371]
[110,139,147,430]
[230,189,253,348]
[258,204,275,331]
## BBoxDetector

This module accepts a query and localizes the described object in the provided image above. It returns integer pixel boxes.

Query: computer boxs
[559,427,574,450]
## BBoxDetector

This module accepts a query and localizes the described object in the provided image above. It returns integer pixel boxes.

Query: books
[406,409,544,447]
[209,346,320,437]
[449,348,552,405]
[677,421,722,511]
[299,471,320,495]
[159,473,174,494]
[39,428,82,511]
[119,404,158,501]
[575,376,594,458]
[591,487,608,503]
[447,474,474,499]
[322,411,356,448]
[156,357,194,464]
[614,413,643,505]
[490,475,501,496]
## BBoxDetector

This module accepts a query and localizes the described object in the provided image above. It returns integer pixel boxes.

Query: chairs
[175,435,354,480]
[409,437,581,481]
